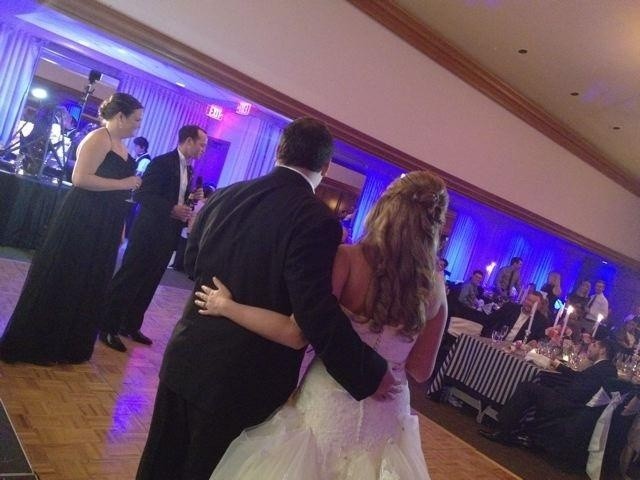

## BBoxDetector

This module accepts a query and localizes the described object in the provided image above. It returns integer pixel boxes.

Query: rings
[203,303,206,309]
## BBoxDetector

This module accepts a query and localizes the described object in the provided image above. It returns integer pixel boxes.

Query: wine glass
[500,325,509,341]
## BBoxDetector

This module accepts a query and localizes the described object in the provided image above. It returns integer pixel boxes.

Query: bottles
[184,188,197,209]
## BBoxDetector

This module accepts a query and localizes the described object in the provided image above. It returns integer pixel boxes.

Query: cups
[492,330,501,342]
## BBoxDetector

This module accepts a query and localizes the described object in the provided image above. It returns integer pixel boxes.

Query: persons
[125,137,150,239]
[97,125,208,352]
[194,168,450,480]
[129,115,401,480]
[0,93,144,367]
[457,257,640,480]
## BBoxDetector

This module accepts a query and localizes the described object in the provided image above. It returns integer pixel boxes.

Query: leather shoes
[479,427,540,449]
[98,331,128,353]
[118,328,153,346]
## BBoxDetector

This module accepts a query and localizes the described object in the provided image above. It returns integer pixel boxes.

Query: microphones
[189,176,203,211]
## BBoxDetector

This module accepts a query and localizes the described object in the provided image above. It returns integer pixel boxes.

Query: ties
[508,270,514,289]
[588,294,598,309]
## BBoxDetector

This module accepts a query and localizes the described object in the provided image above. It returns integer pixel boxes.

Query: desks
[1,169,138,253]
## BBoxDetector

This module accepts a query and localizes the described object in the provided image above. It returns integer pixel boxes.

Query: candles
[528,301,538,330]
[591,320,600,337]
[553,303,569,338]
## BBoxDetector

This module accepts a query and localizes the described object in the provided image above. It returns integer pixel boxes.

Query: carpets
[1,393,35,477]
[403,372,593,480]
